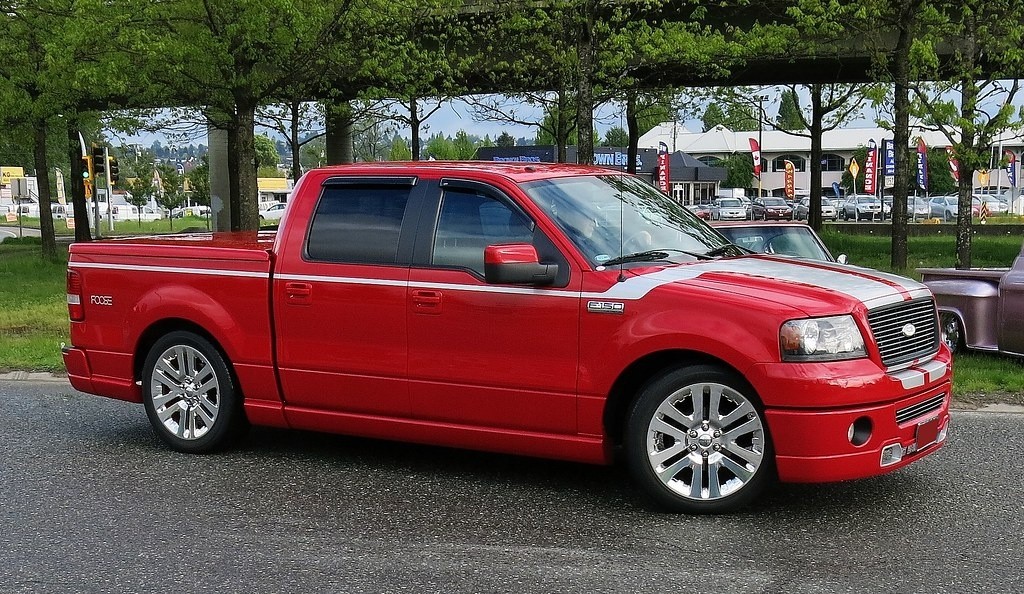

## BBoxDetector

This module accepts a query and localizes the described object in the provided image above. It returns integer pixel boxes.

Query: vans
[12,201,212,223]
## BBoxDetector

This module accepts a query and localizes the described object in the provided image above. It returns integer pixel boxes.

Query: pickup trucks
[64,163,953,513]
[913,240,1024,358]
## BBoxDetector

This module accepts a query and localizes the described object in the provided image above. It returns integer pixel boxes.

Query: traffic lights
[108,159,120,182]
[93,146,106,175]
[82,156,91,181]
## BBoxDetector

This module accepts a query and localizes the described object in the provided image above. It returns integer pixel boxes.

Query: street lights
[752,94,770,198]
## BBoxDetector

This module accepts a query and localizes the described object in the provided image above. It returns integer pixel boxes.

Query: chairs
[436,182,532,270]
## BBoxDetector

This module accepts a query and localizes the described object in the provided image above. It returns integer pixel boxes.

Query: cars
[706,221,849,265]
[259,203,287,220]
[686,194,1013,222]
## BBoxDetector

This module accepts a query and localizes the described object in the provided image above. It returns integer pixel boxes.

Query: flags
[659,137,1016,200]
